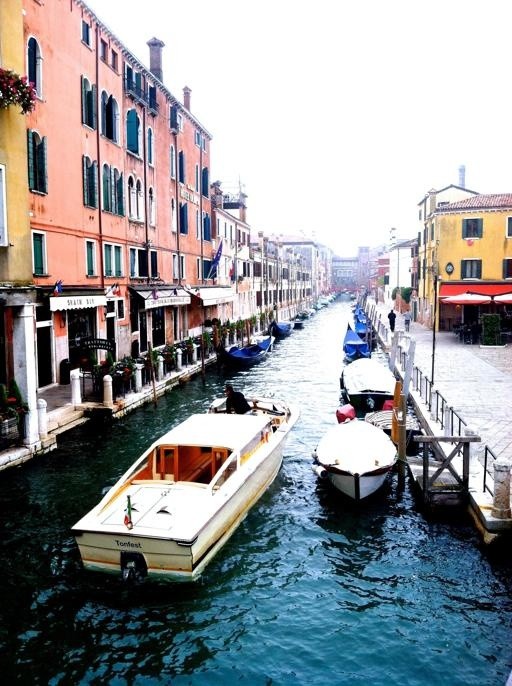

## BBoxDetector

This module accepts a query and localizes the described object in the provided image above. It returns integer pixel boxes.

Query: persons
[225,383,251,415]
[402,309,413,331]
[387,310,396,332]
[454,319,482,344]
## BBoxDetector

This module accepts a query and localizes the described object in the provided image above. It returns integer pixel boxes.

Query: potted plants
[91,319,244,382]
[1,379,30,425]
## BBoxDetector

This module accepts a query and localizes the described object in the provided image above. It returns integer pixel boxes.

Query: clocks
[446,262,454,275]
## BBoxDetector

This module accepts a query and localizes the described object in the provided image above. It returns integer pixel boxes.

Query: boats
[271,323,292,340]
[68,395,303,587]
[310,402,399,505]
[357,311,371,324]
[342,322,370,362]
[355,321,374,339]
[223,334,276,366]
[340,357,396,410]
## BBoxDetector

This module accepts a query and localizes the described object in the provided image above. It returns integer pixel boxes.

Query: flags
[52,280,59,297]
[173,287,178,296]
[111,282,118,296]
[211,239,223,267]
[116,282,122,297]
[152,287,158,301]
[57,279,63,294]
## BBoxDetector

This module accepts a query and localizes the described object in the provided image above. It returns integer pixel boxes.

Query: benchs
[156,446,222,482]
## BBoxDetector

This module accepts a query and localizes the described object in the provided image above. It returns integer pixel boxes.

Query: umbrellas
[493,293,512,305]
[439,290,491,323]
